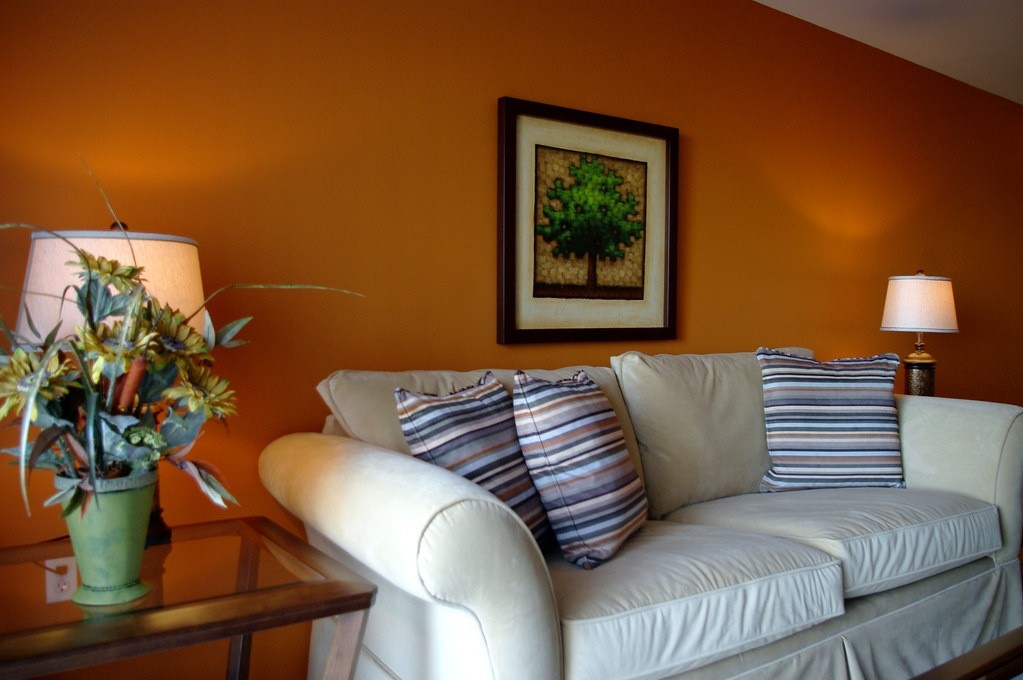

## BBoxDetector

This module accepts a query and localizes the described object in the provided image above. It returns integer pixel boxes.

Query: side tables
[0,515,378,680]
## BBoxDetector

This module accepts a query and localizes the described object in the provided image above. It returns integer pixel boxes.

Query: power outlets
[44,556,78,604]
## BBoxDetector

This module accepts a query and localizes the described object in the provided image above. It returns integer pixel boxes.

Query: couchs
[256,348,1023,680]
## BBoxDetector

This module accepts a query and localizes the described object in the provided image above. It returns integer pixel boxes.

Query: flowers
[0,169,367,520]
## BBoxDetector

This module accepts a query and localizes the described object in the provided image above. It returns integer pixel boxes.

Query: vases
[54,464,159,608]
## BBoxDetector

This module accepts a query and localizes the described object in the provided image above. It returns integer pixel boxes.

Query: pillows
[394,370,551,545]
[513,368,649,570]
[755,346,906,494]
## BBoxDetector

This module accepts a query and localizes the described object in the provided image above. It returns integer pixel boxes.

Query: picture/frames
[496,95,678,347]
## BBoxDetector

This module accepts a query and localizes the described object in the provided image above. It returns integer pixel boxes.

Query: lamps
[879,269,960,397]
[12,222,206,550]
